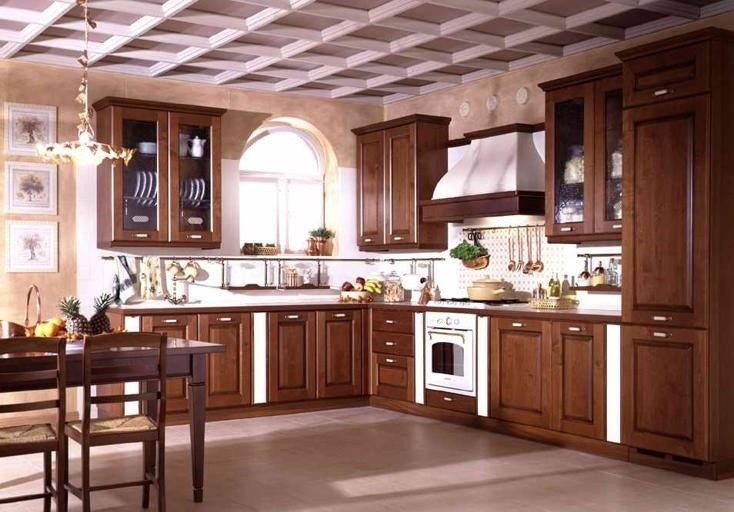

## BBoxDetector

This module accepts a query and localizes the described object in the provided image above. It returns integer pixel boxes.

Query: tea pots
[187,136,206,157]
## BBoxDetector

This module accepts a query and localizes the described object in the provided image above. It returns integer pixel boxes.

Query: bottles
[284,267,314,286]
[576,258,622,287]
[533,273,562,300]
[384,271,405,301]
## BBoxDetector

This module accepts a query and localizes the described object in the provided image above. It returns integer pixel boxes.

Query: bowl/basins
[340,290,371,299]
[138,142,156,153]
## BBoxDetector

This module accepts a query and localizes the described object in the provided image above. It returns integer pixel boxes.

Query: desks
[2,329,227,504]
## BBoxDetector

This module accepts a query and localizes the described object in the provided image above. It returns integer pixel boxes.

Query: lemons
[34,322,59,338]
[47,317,64,329]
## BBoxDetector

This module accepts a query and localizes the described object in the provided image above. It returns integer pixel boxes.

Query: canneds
[266,244,274,247]
[244,243,254,255]
[254,243,262,255]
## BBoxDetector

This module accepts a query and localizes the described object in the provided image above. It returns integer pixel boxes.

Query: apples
[356,277,365,285]
[342,282,353,291]
[354,283,364,291]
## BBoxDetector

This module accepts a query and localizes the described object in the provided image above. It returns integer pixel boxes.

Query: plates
[181,176,206,206]
[132,171,157,206]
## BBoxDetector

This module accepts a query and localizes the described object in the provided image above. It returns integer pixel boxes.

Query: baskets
[528,297,580,310]
[243,247,278,255]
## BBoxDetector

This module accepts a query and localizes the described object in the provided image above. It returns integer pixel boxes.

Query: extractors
[421,124,546,224]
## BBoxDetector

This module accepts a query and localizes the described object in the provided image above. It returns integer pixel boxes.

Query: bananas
[363,279,384,294]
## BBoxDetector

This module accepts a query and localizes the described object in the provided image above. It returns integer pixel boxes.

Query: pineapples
[89,292,115,335]
[55,297,93,340]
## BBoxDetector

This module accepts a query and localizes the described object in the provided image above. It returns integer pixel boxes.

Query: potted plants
[310,229,333,256]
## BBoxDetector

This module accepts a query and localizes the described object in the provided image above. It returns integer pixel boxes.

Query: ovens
[423,329,479,398]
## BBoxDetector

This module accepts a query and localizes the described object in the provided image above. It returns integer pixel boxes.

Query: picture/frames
[3,160,59,215]
[5,218,60,274]
[3,101,59,158]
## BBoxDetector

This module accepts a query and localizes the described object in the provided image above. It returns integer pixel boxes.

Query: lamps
[39,0,136,175]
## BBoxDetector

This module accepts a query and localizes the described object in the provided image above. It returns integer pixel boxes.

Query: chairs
[1,333,69,512]
[62,331,174,512]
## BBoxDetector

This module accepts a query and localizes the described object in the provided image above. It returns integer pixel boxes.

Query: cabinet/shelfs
[534,62,624,244]
[267,304,371,418]
[613,24,733,483]
[368,301,414,417]
[352,113,452,253]
[96,305,253,426]
[489,305,607,457]
[91,94,229,250]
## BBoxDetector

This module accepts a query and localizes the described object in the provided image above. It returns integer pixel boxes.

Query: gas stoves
[427,296,518,310]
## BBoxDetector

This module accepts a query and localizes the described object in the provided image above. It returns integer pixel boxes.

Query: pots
[466,274,505,300]
